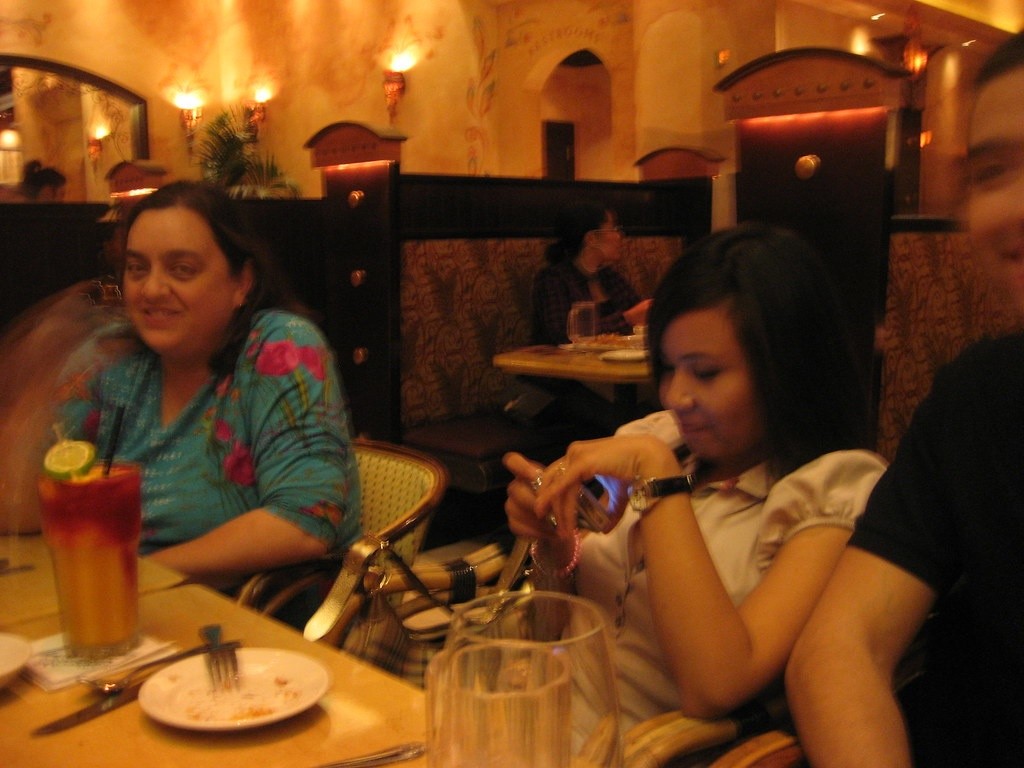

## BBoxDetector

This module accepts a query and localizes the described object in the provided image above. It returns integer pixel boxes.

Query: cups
[33,459,145,660]
[423,592,622,768]
[633,326,647,334]
[567,302,596,342]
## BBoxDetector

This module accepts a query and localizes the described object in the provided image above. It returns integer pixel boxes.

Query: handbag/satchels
[305,530,518,688]
[0,277,139,535]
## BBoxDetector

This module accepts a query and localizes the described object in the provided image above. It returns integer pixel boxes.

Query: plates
[599,349,646,360]
[559,335,635,350]
[139,649,328,732]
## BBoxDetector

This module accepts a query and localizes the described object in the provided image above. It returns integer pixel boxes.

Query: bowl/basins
[0,633,31,689]
[624,335,649,350]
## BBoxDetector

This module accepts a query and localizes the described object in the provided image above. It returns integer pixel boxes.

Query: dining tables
[0,534,425,768]
[493,345,653,384]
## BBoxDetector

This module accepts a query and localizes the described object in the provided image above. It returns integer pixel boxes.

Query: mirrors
[0,53,150,203]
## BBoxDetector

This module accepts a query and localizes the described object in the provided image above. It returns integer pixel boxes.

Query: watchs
[626,474,690,512]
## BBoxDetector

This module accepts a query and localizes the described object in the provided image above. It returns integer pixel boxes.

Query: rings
[558,461,567,474]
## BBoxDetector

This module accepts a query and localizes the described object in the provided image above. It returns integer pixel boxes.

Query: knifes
[31,679,146,735]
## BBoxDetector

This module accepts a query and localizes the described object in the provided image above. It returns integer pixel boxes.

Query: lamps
[243,84,273,145]
[175,90,204,156]
[383,68,405,117]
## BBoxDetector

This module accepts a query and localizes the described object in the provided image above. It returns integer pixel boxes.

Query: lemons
[43,440,97,477]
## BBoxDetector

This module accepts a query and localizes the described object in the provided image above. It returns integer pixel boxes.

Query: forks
[198,624,239,691]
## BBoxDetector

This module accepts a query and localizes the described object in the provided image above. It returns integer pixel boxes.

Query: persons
[527,186,713,498]
[19,159,67,202]
[782,28,1024,768]
[483,220,887,768]
[21,179,365,583]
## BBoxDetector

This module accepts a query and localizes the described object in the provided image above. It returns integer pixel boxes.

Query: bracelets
[531,531,579,577]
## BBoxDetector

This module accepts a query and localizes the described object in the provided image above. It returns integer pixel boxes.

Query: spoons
[76,640,240,694]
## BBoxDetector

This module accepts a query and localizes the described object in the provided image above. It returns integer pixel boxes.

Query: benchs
[321,159,714,492]
[873,216,1023,463]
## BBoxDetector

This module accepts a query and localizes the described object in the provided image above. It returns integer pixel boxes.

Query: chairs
[234,437,449,618]
